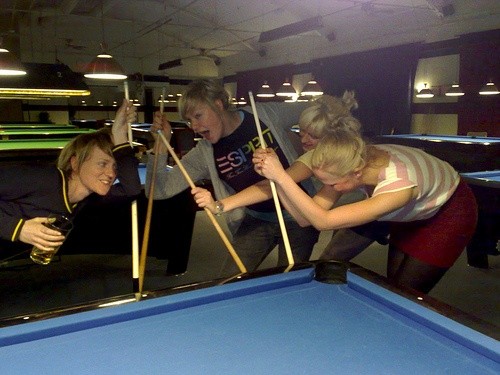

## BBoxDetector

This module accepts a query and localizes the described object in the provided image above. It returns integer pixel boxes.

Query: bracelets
[216,201,224,217]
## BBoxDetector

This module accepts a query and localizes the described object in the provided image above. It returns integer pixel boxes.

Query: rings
[260,159,264,165]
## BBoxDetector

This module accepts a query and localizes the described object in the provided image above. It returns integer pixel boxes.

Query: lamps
[0,0,141,107]
[300,39,324,96]
[275,38,296,96]
[479,83,500,94]
[445,56,465,96]
[157,41,247,105]
[416,44,435,98]
[257,42,275,98]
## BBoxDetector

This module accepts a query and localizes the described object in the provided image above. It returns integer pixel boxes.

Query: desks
[0,120,217,274]
[0,259,500,375]
[377,134,500,174]
[459,171,500,269]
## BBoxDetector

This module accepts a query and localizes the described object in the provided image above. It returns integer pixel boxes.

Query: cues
[124,82,140,294]
[139,87,167,293]
[157,129,247,274]
[248,91,294,264]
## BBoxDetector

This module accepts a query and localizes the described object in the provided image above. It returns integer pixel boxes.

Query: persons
[253,116,477,294]
[191,89,390,260]
[0,98,143,260]
[144,76,320,279]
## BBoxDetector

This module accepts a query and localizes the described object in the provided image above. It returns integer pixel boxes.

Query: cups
[30,214,73,264]
